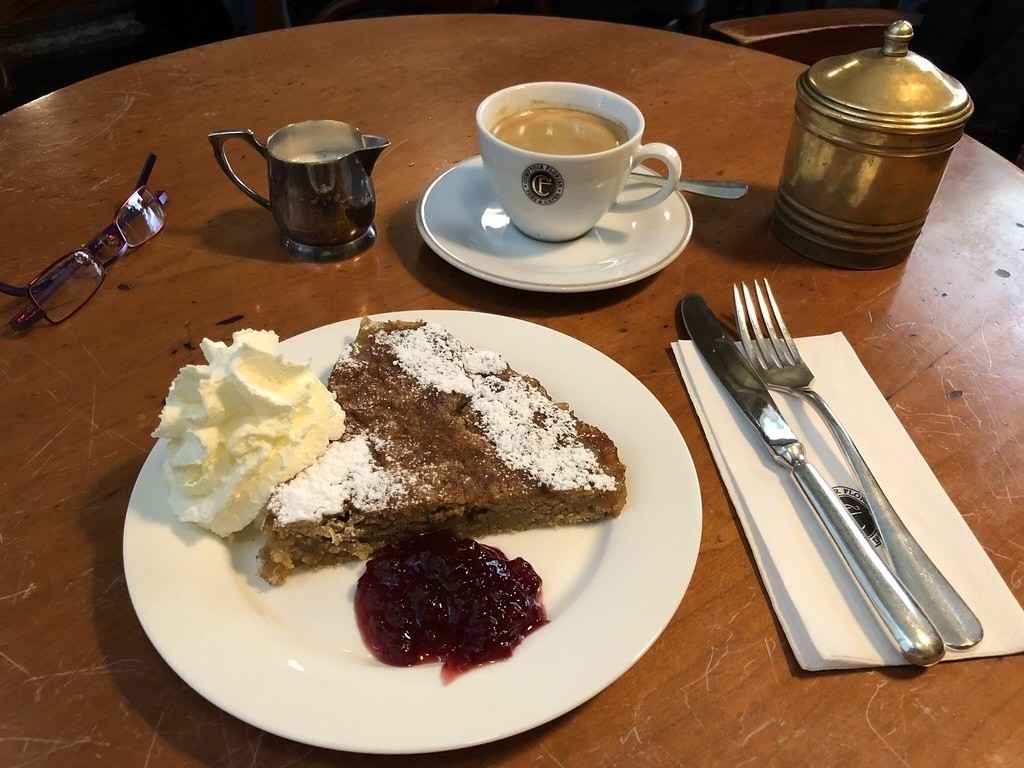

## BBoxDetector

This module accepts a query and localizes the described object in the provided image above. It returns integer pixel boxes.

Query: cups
[772,21,972,270]
[474,82,682,242]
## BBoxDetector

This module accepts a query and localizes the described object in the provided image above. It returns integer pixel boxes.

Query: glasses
[0,152,168,332]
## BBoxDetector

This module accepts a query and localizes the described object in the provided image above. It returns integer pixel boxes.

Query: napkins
[670,328,1024,672]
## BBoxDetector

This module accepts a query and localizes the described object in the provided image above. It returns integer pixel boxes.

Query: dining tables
[0,13,1024,768]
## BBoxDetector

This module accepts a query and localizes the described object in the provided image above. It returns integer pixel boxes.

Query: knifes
[682,292,946,667]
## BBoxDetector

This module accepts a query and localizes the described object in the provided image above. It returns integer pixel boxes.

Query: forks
[733,277,984,651]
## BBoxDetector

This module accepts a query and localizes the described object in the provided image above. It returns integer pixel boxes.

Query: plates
[122,309,702,754]
[415,156,693,293]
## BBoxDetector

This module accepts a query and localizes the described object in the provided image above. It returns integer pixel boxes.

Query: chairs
[707,8,903,67]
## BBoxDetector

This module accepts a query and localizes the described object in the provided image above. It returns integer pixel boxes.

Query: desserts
[149,313,628,588]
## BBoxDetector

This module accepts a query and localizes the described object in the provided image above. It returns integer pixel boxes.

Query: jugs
[206,120,392,263]
